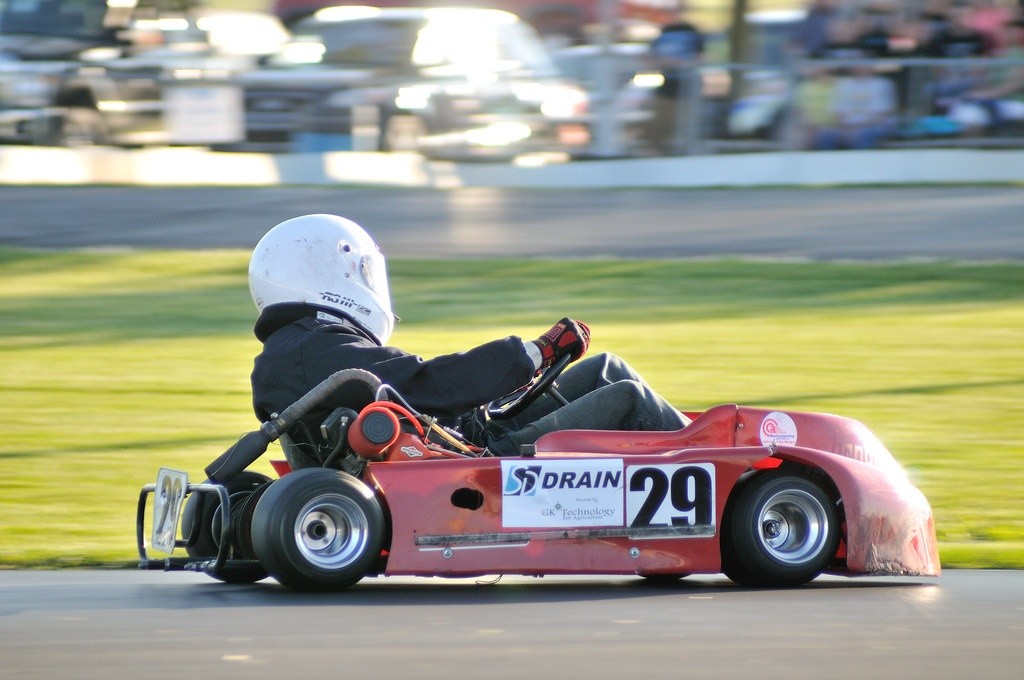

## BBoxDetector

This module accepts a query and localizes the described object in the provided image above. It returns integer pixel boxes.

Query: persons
[246,212,691,464]
[723,0,1023,153]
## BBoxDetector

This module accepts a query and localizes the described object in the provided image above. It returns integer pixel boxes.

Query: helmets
[248,213,394,346]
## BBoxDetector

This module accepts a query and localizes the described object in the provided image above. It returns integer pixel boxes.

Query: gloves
[531,317,591,378]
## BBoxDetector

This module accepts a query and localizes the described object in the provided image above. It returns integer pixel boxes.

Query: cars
[0,7,1024,161]
